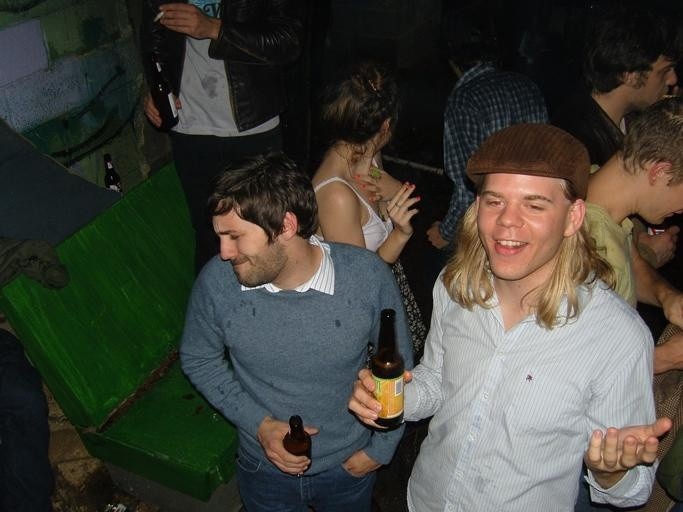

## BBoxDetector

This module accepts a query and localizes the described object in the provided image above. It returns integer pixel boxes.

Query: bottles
[280,416,312,473]
[102,155,122,192]
[147,58,181,131]
[370,310,406,430]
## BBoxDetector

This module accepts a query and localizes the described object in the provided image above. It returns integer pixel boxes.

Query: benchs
[1,163,242,512]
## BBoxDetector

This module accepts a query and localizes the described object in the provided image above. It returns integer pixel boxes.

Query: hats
[464,122,590,199]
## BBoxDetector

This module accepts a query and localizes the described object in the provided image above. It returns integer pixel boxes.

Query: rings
[394,202,400,209]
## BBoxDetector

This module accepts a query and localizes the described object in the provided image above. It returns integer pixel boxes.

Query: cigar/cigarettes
[153,11,165,24]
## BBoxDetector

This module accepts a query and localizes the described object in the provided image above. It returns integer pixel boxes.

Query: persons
[575,94,682,511]
[137,0,318,277]
[344,121,672,512]
[306,56,428,371]
[550,0,683,270]
[179,143,413,512]
[424,0,548,300]
